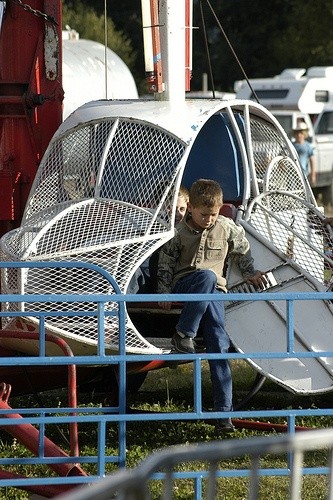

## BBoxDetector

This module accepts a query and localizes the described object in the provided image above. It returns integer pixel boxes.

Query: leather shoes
[171,331,194,354]
[218,418,235,432]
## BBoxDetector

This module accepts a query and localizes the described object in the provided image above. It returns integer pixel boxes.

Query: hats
[292,122,309,131]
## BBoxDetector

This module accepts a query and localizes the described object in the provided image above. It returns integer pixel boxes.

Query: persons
[282,122,316,208]
[156,180,268,431]
[79,184,192,307]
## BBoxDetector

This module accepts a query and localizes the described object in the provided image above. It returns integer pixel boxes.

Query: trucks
[234,66,333,204]
